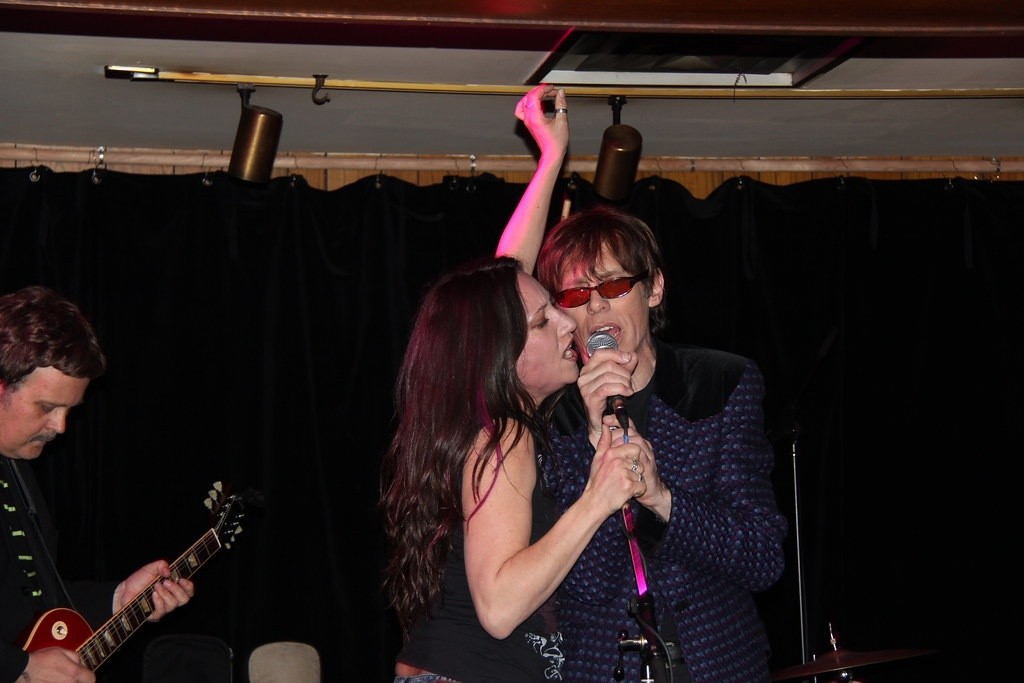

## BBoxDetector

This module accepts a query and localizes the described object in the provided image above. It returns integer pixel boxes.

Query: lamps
[227,84,284,182]
[592,97,642,199]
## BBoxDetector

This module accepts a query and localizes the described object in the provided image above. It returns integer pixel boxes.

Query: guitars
[40,479,249,675]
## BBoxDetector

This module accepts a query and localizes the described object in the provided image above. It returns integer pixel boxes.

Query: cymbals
[768,644,947,682]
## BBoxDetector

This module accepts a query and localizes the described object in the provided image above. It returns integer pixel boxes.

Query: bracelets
[632,459,637,470]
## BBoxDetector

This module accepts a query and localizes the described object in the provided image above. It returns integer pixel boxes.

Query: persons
[385,83,646,683]
[536,206,785,683]
[0,284,194,683]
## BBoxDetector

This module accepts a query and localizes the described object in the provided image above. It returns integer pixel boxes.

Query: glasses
[552,270,650,309]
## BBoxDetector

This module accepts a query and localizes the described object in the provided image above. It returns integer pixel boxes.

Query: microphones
[587,331,630,431]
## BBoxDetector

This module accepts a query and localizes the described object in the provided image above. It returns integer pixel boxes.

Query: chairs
[142,634,234,683]
[248,642,321,683]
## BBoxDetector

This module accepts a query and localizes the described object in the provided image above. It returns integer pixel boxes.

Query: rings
[639,474,643,481]
[554,109,567,113]
